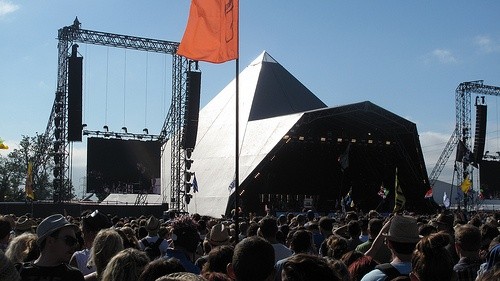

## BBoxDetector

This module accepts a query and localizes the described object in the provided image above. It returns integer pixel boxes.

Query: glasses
[51,234,78,247]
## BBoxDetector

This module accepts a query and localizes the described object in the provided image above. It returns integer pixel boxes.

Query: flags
[25,163,34,200]
[177,0,239,64]
[395,175,406,212]
[379,187,389,198]
[460,177,470,192]
[424,189,433,198]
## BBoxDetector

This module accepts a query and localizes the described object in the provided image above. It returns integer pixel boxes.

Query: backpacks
[140,237,164,261]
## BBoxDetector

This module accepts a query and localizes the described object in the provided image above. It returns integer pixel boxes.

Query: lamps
[282,134,394,146]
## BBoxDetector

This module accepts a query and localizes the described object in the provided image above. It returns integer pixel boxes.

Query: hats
[14,215,35,237]
[206,224,232,246]
[144,215,160,233]
[382,214,426,243]
[432,213,454,228]
[36,214,78,246]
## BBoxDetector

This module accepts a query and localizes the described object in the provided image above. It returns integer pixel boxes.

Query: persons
[139,216,168,258]
[432,213,454,234]
[318,217,332,256]
[356,219,391,262]
[196,224,230,268]
[19,214,85,281]
[362,215,423,281]
[347,221,364,248]
[0,205,500,281]
[259,218,292,263]
[70,210,113,276]
